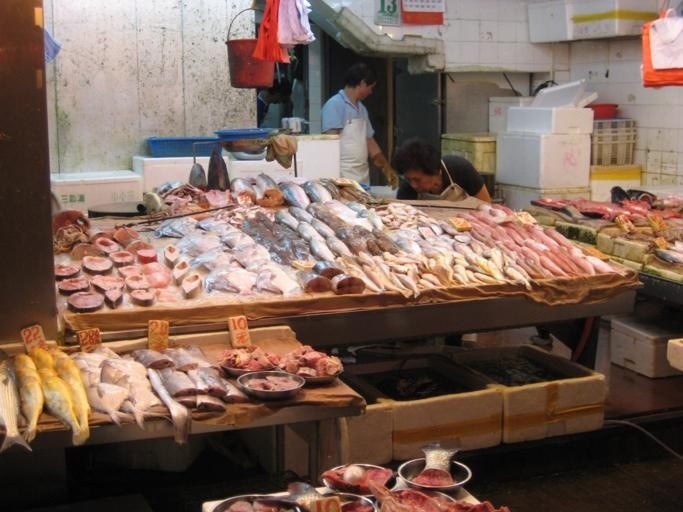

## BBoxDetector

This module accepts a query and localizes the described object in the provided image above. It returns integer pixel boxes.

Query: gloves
[370,152,400,190]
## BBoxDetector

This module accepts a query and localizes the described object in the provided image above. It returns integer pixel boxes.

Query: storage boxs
[282,317,680,481]
[52,128,340,216]
[440,79,682,217]
[526,0,664,45]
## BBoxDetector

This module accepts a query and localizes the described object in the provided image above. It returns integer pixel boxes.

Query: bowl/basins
[280,118,305,133]
[217,349,344,398]
[213,458,471,512]
[215,128,275,151]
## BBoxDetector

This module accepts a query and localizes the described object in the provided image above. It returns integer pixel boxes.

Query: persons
[257,77,292,128]
[395,138,491,348]
[320,62,400,190]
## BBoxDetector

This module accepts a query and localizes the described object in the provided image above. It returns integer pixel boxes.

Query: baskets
[144,135,224,156]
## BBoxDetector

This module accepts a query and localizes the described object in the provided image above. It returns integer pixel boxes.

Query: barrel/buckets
[147,135,222,158]
[225,5,276,88]
[214,126,276,142]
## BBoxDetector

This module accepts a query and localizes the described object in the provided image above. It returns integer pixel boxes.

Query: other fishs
[0,346,192,451]
[654,239,683,263]
[154,175,628,300]
